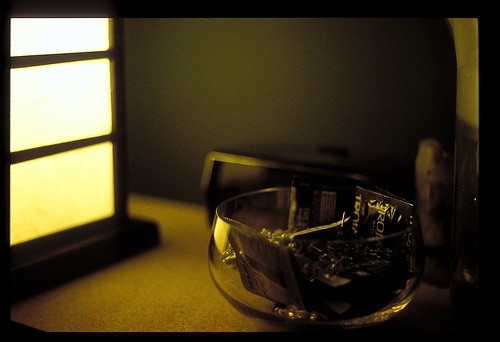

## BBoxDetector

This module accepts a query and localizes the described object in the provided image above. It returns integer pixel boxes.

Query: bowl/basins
[205,187,426,328]
